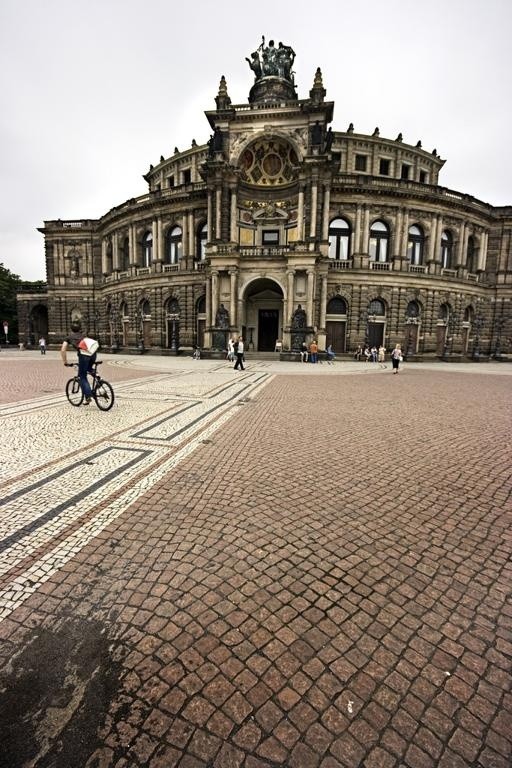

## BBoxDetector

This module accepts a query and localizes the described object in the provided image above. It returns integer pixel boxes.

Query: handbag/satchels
[78,337,100,356]
[391,354,394,358]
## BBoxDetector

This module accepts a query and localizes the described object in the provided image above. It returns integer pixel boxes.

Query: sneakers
[98,378,103,387]
[83,399,92,404]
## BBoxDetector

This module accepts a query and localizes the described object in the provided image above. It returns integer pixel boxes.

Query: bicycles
[64,360,115,411]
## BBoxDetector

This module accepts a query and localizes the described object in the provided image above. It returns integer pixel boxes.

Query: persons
[39,336,47,355]
[228,340,236,362]
[260,35,285,76]
[62,321,102,405]
[352,345,362,361]
[300,342,309,363]
[378,344,386,362]
[216,303,230,329]
[389,343,402,374]
[233,336,246,370]
[309,340,318,363]
[364,345,372,362]
[326,344,336,361]
[371,345,378,362]
[290,303,307,328]
